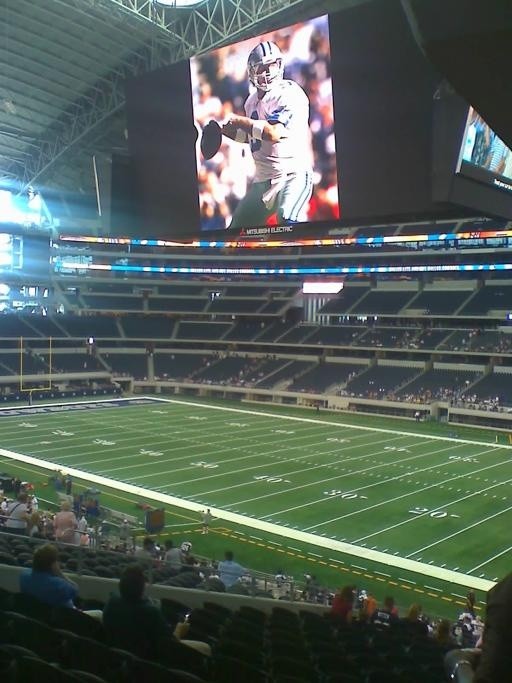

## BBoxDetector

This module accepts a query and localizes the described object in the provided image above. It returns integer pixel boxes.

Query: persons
[338,324,512,412]
[1,467,512,682]
[462,109,508,175]
[415,411,421,423]
[187,23,339,231]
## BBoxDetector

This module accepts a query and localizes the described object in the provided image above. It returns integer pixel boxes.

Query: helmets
[247,40,285,92]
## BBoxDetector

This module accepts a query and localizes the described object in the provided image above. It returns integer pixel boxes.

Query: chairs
[0,529,464,683]
